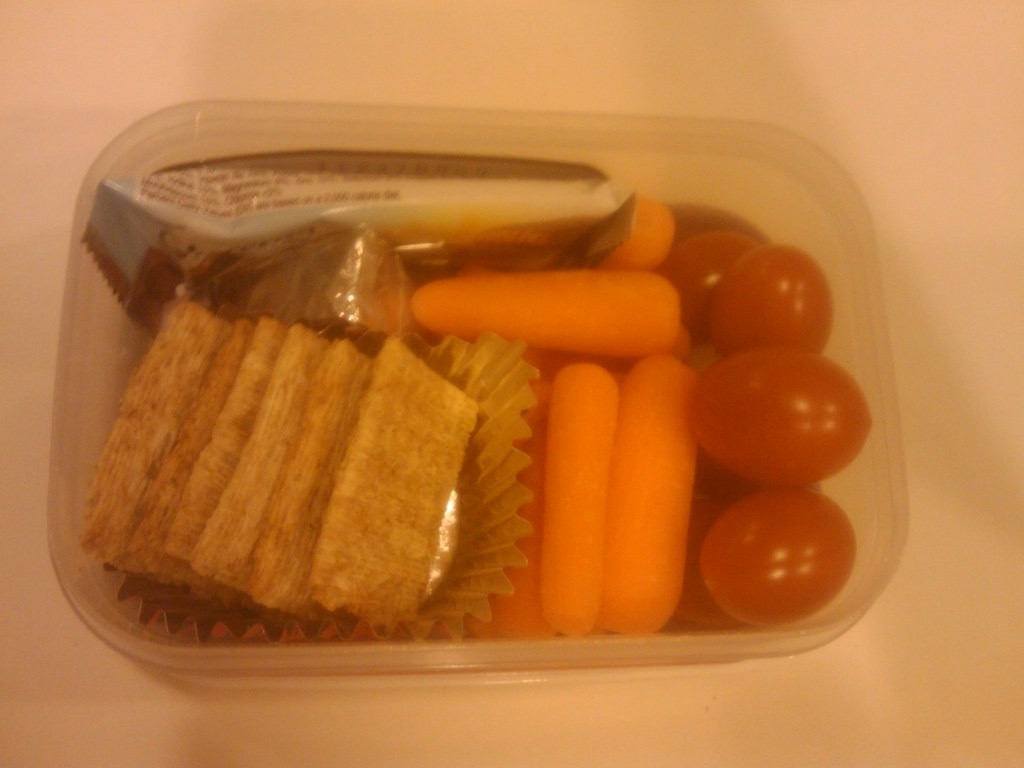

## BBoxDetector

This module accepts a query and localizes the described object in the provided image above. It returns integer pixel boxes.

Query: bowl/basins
[48,100,908,689]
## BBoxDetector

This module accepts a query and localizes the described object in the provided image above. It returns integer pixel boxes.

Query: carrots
[410,200,700,637]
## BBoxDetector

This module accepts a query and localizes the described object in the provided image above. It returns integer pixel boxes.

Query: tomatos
[672,231,874,626]
